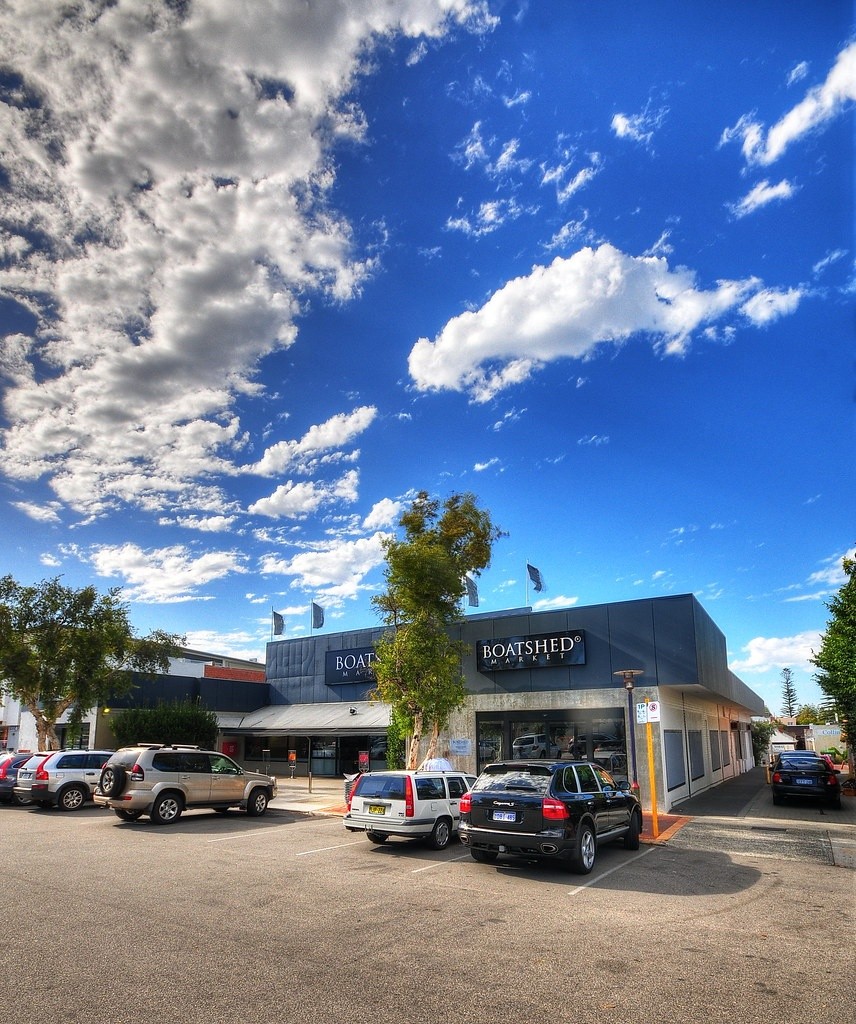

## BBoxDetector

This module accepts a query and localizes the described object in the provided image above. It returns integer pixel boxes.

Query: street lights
[613,669,645,802]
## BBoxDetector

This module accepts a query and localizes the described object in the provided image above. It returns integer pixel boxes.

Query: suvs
[12,747,117,811]
[457,759,643,876]
[344,768,477,850]
[0,749,41,807]
[93,742,278,825]
[568,731,627,760]
[513,733,563,760]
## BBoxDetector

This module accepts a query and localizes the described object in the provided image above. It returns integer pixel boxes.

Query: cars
[370,741,387,760]
[311,741,337,757]
[769,748,841,810]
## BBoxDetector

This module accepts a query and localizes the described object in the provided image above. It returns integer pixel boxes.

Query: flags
[527,564,542,593]
[312,602,325,629]
[273,611,284,635]
[464,575,478,607]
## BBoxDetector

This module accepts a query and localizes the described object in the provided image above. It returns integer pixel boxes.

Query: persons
[514,748,529,758]
[417,749,452,771]
[531,745,543,758]
